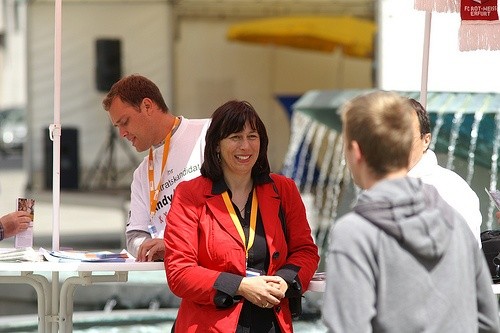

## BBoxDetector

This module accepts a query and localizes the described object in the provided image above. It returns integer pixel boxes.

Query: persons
[103,75,212,263]
[496,211,500,219]
[322,92,500,333]
[0,211,30,242]
[163,100,320,333]
[406,98,483,249]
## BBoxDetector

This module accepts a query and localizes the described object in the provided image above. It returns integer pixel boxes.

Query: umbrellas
[221,15,375,88]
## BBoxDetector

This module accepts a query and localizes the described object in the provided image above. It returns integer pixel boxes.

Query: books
[484,187,500,211]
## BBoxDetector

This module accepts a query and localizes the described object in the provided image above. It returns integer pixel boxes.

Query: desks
[0,248,166,333]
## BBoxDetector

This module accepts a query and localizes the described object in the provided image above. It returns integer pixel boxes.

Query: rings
[264,302,269,307]
